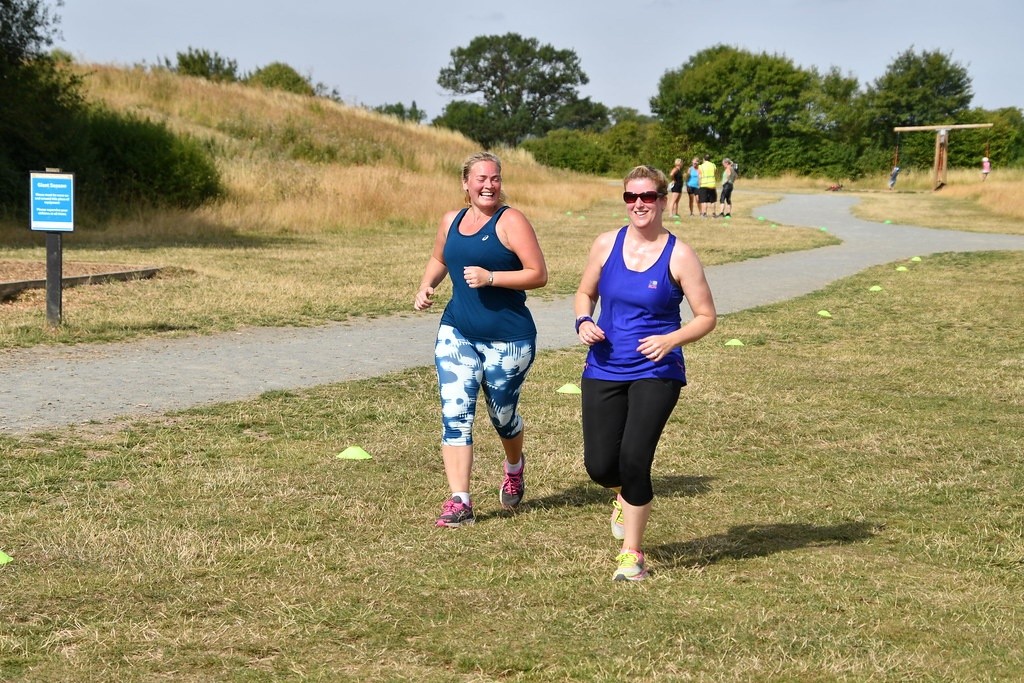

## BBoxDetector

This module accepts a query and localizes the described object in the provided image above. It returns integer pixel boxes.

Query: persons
[717,158,738,218]
[982,157,990,174]
[685,158,702,217]
[414,152,548,528]
[575,165,717,582]
[698,154,717,218]
[670,158,684,219]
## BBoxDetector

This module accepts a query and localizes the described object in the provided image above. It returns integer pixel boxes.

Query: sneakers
[610,548,650,583]
[609,494,625,541]
[434,495,475,529]
[499,452,525,510]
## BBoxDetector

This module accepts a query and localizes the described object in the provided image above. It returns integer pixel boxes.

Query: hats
[692,159,699,165]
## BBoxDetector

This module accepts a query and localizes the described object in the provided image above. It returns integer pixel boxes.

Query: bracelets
[489,271,493,286]
[575,315,594,335]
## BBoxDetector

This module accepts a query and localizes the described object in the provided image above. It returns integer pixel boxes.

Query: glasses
[623,191,665,204]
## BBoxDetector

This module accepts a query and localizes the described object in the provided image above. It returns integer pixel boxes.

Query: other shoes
[690,213,694,217]
[669,214,680,218]
[699,213,704,218]
[712,213,717,218]
[703,212,708,217]
[716,212,724,218]
[724,213,731,218]
